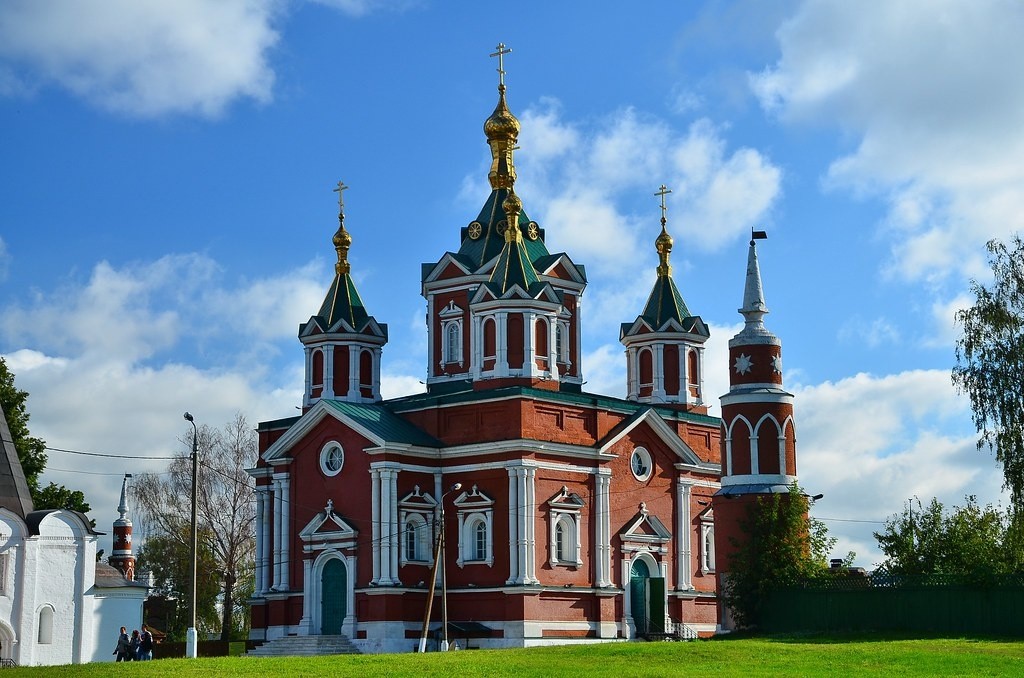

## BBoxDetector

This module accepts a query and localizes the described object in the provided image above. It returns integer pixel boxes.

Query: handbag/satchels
[131,638,140,652]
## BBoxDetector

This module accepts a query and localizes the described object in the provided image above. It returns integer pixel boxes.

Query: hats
[133,630,139,636]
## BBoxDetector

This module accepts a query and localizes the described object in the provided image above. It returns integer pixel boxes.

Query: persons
[137,624,152,660]
[126,629,141,661]
[113,627,131,662]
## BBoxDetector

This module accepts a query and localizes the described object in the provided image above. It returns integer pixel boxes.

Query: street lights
[183,412,198,659]
[441,482,463,651]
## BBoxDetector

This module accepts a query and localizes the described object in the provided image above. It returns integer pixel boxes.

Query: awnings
[437,622,491,633]
